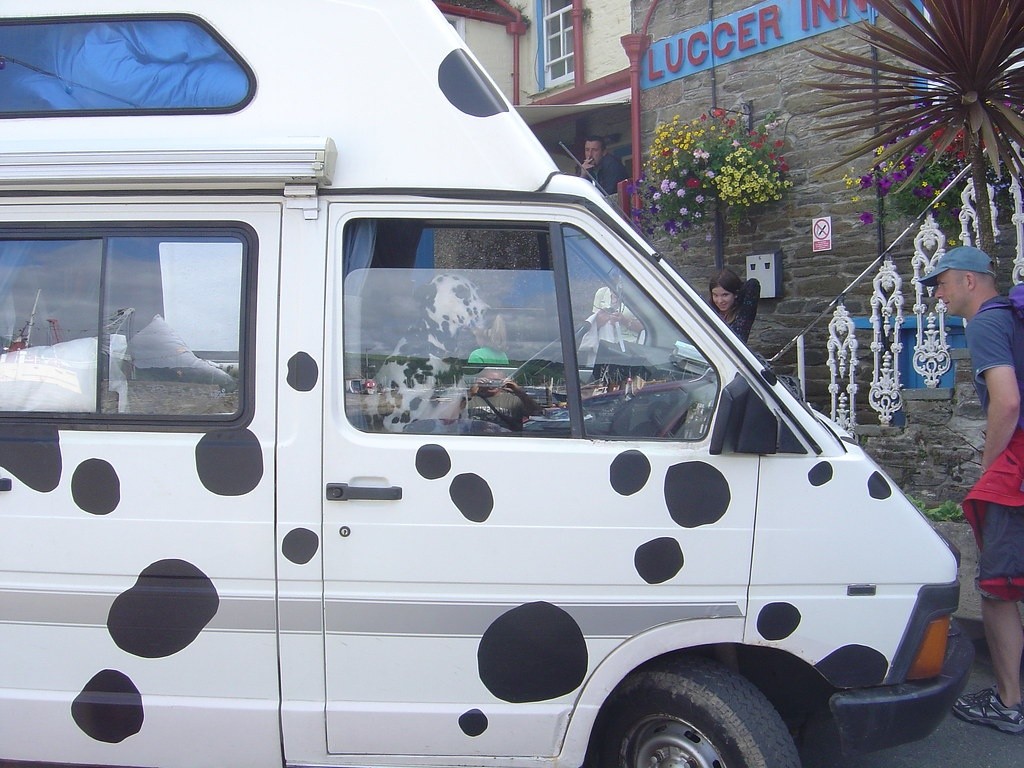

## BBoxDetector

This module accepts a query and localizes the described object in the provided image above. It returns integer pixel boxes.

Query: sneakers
[951,683,1024,735]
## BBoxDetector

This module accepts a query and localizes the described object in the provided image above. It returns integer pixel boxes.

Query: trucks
[1,0,977,767]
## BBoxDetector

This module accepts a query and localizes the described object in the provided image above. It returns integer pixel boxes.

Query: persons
[916,246,1024,733]
[706,269,760,344]
[575,135,628,197]
[588,287,649,393]
[440,370,545,435]
[466,313,510,366]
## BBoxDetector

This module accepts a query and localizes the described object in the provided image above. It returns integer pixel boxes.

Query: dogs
[363,273,497,434]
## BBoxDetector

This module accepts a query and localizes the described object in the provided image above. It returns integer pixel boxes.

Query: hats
[917,245,997,287]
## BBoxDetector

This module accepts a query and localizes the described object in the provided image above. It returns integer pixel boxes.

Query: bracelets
[627,320,633,327]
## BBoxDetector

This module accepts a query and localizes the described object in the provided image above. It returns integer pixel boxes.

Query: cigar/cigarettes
[584,159,589,162]
